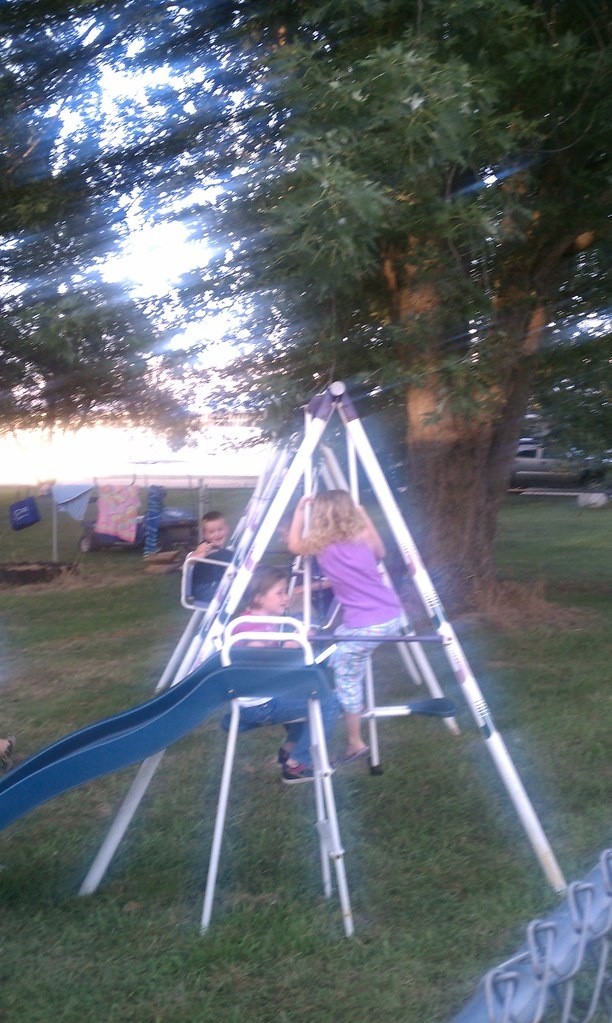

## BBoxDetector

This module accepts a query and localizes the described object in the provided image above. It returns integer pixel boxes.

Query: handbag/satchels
[11,485,41,531]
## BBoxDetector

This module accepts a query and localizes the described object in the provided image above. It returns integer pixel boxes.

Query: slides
[0,647,329,836]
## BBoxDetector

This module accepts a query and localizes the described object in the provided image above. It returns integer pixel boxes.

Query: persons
[218,560,342,785]
[190,511,258,625]
[278,489,406,766]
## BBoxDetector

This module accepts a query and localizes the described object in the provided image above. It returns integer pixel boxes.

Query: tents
[23,417,316,565]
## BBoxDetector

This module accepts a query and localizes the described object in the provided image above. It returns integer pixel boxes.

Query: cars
[510,436,612,487]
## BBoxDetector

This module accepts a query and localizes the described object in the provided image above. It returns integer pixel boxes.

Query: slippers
[339,704,365,719]
[342,746,369,765]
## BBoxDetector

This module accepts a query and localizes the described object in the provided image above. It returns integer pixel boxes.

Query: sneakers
[278,747,290,764]
[281,765,315,785]
